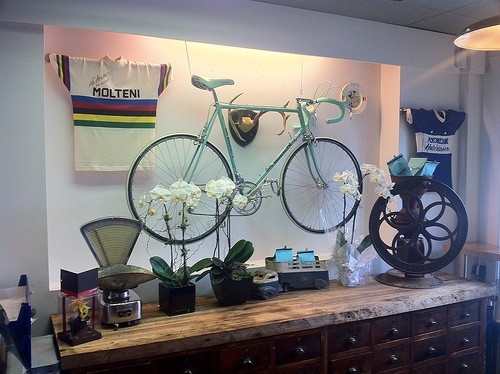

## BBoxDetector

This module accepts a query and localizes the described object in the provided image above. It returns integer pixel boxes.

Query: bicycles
[124,74,364,246]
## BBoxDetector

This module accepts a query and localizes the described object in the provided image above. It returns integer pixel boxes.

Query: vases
[335,260,366,287]
[209,273,253,305]
[159,281,196,317]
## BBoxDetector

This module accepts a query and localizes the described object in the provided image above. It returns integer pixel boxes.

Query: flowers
[139,178,202,287]
[334,164,395,260]
[205,176,254,284]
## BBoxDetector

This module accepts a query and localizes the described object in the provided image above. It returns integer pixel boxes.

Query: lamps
[453,15,500,51]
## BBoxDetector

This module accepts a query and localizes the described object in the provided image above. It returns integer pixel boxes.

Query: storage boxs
[60,265,98,298]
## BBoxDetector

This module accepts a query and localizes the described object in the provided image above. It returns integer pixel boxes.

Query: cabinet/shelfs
[50,270,497,374]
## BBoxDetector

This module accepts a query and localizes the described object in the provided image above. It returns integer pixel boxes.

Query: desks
[444,241,500,282]
